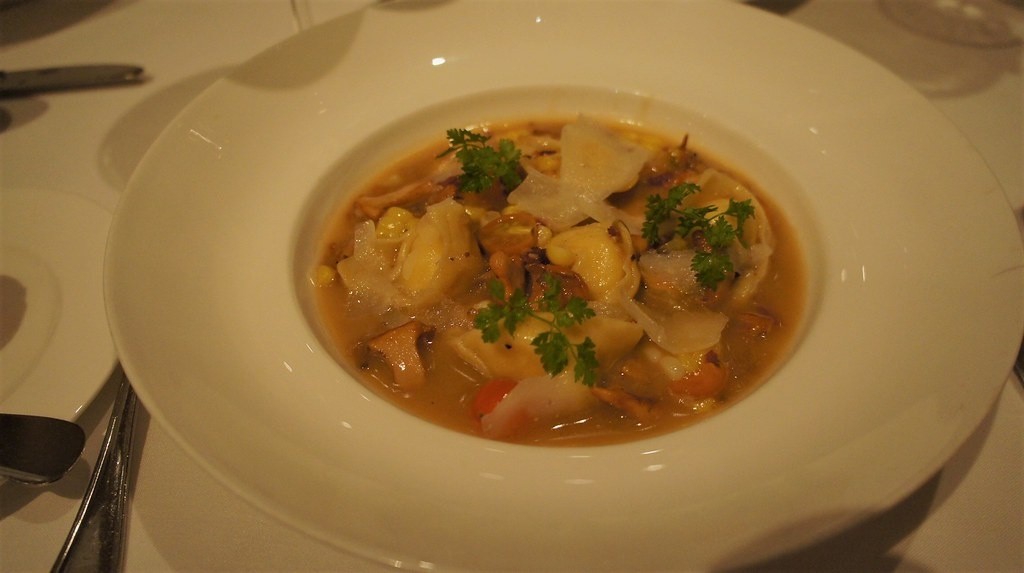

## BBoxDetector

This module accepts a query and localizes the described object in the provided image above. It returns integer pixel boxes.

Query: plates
[0,188,117,483]
[103,0,1024,573]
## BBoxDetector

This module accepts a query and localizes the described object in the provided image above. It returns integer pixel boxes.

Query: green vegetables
[433,128,758,388]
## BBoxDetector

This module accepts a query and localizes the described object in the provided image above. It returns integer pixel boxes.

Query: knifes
[0,64,146,95]
